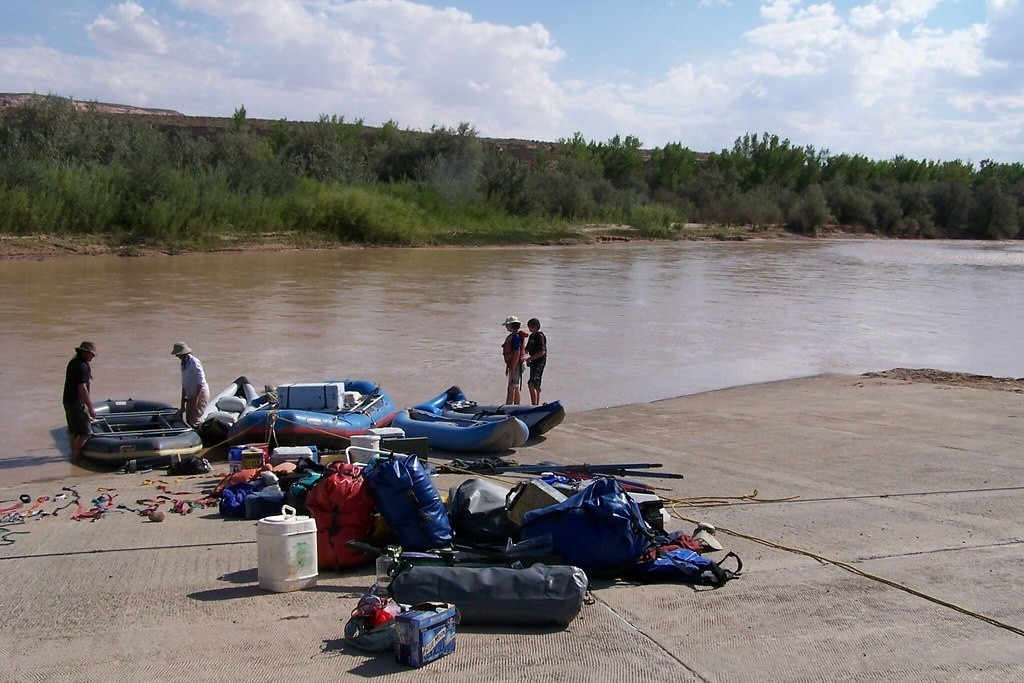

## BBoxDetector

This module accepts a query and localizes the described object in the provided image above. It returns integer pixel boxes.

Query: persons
[501,315,527,405]
[61,340,102,462]
[524,317,548,405]
[169,340,210,429]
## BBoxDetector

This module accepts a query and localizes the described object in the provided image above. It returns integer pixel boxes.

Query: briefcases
[394,602,457,669]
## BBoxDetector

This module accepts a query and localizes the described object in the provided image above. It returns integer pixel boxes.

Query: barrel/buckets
[350,435,382,466]
[257,505,320,592]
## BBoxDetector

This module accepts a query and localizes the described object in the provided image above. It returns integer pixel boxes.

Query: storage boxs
[277,382,345,411]
[382,437,429,463]
[228,449,243,463]
[272,445,314,468]
[366,427,406,439]
[243,448,264,468]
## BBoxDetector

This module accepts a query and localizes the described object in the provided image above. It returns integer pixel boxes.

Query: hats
[502,316,521,326]
[170,342,192,355]
[75,341,99,356]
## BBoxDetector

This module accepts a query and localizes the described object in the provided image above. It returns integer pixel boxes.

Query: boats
[77,396,202,468]
[388,406,527,456]
[230,382,397,448]
[419,384,567,441]
[200,374,256,436]
